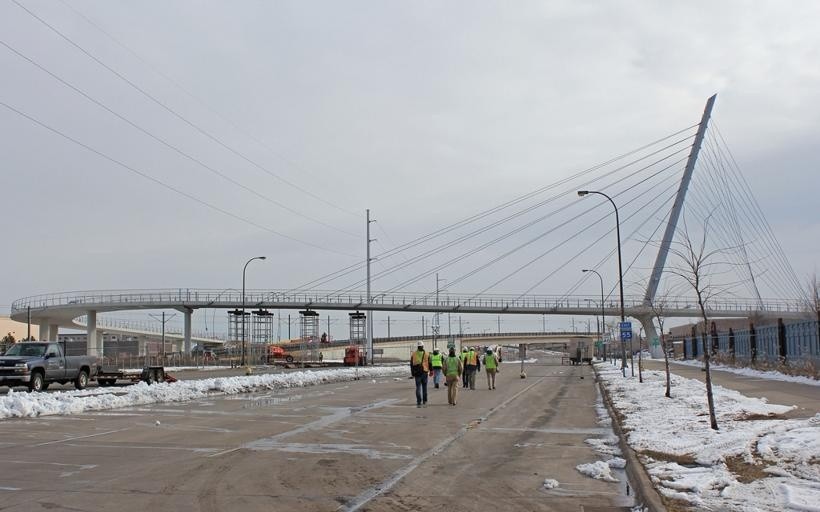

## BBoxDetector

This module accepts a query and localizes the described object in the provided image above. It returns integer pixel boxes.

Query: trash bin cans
[141,366,164,384]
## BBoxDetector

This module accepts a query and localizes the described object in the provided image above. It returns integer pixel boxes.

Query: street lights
[241,256,266,365]
[582,269,607,361]
[577,190,626,379]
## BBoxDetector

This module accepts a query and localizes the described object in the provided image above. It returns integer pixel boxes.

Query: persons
[319,351,323,362]
[245,366,252,375]
[409,340,434,408]
[429,345,499,406]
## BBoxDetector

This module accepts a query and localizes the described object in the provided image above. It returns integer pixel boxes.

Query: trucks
[0,339,99,393]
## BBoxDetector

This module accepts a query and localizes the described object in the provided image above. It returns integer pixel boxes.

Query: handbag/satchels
[411,364,423,377]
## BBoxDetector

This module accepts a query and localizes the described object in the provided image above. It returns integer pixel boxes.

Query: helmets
[416,341,494,352]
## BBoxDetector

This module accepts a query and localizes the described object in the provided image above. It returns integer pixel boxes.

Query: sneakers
[463,383,468,388]
[435,383,439,388]
[417,401,425,405]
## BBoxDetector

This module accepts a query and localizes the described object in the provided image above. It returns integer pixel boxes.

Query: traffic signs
[620,322,632,339]
